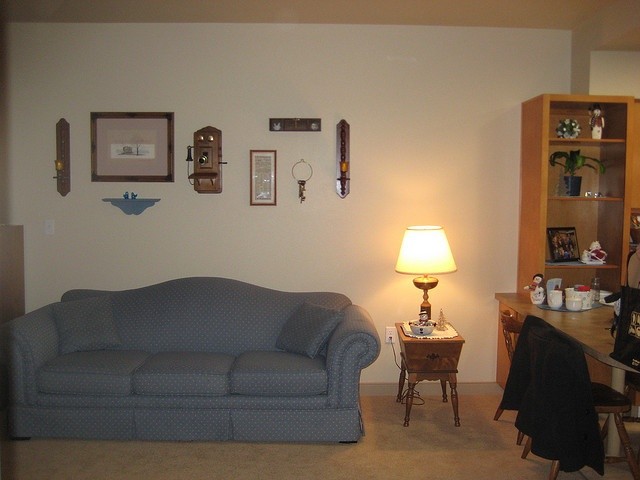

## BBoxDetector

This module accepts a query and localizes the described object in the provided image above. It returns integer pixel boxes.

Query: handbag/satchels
[604,250,640,373]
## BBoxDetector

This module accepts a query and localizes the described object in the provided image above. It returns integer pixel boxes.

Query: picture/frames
[248,148,278,207]
[88,109,175,184]
[546,225,581,263]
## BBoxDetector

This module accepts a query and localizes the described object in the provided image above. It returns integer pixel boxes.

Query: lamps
[393,222,458,325]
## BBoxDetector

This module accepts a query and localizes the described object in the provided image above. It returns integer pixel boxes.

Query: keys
[294,183,310,205]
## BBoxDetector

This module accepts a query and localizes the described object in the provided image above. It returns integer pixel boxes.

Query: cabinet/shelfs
[492,287,639,458]
[514,91,640,299]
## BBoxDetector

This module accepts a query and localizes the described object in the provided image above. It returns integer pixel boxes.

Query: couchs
[0,275,381,447]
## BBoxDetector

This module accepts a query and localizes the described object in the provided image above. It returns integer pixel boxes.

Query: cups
[565,287,583,311]
[547,290,562,310]
[582,289,593,310]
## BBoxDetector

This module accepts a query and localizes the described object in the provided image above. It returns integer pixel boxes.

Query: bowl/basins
[409,320,436,336]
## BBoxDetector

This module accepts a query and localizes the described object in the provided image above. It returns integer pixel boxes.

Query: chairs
[517,313,639,478]
[493,309,588,445]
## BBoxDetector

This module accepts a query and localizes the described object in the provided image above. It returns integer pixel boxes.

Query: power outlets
[384,325,397,345]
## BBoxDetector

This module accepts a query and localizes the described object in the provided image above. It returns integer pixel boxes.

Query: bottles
[590,275,600,301]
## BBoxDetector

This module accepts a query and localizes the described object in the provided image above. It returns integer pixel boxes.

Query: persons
[581,240,608,265]
[548,228,579,259]
[524,274,546,305]
[588,104,605,139]
[409,311,438,336]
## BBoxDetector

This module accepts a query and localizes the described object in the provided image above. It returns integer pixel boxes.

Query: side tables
[387,319,465,428]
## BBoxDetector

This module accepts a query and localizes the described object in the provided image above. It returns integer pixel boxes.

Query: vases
[548,146,605,199]
